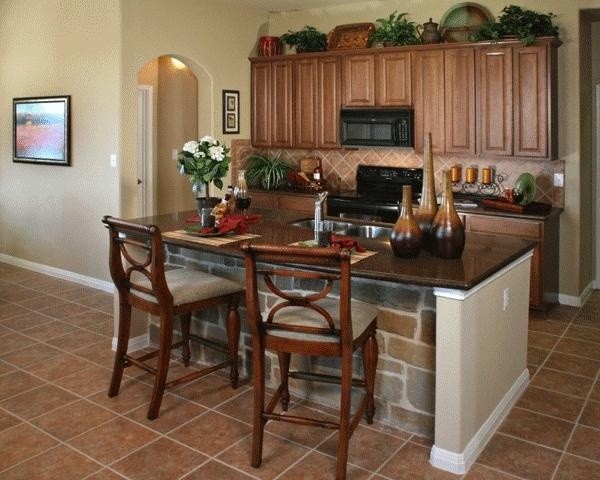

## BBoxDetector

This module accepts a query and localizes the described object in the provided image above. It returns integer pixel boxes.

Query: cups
[201,207,216,229]
[318,219,334,247]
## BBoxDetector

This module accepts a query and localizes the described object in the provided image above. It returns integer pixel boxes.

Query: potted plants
[241,149,295,192]
[278,24,328,55]
[367,9,421,47]
[467,4,560,49]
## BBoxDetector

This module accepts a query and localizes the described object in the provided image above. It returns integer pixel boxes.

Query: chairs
[238,240,382,480]
[101,214,246,422]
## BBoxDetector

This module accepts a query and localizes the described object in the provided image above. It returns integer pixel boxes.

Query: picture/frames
[221,88,242,135]
[10,93,73,168]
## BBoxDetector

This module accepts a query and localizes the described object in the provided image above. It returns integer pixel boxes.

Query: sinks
[289,218,352,235]
[335,225,393,245]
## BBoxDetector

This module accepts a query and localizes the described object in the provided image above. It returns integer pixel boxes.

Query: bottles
[313,159,322,186]
[233,170,249,215]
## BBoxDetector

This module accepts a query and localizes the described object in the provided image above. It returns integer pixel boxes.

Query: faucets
[314,190,329,241]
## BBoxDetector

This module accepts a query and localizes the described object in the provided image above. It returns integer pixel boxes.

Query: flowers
[175,135,235,200]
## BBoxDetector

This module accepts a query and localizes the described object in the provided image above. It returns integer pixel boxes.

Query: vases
[194,196,223,221]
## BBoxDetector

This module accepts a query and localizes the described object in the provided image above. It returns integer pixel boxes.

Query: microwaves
[339,105,414,146]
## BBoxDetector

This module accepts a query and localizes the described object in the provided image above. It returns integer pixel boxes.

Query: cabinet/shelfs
[398,185,566,313]
[246,187,276,213]
[275,193,327,219]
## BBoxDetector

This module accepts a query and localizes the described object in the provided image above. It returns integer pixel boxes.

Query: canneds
[466,168,475,183]
[482,168,491,184]
[452,166,458,182]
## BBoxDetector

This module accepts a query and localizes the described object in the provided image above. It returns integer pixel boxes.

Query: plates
[185,224,234,236]
[514,171,536,206]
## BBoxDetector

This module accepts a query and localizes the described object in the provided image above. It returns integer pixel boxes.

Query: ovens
[326,204,401,223]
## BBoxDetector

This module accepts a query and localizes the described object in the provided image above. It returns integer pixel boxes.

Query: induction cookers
[328,165,423,206]
